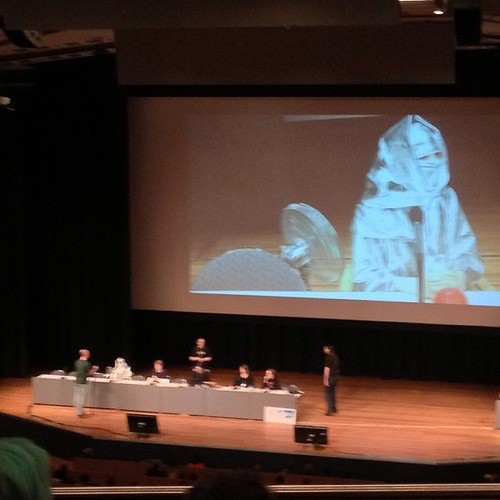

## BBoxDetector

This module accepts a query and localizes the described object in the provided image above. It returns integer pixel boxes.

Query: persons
[187,338,213,385]
[233,364,254,386]
[150,360,172,384]
[261,369,282,390]
[0,436,313,500]
[73,350,99,417]
[342,113,486,296]
[323,343,340,415]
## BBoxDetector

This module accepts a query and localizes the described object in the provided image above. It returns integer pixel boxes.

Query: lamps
[0,96,18,111]
[432,2,448,15]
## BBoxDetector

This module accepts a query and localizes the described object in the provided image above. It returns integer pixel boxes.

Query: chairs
[49,370,66,375]
[191,248,306,291]
[69,372,76,375]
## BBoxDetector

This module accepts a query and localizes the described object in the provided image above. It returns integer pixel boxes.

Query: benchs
[46,456,385,488]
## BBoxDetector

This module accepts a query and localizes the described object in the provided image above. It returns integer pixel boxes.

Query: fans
[280,202,344,293]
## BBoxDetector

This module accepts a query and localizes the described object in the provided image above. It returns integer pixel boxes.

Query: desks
[33,373,306,424]
